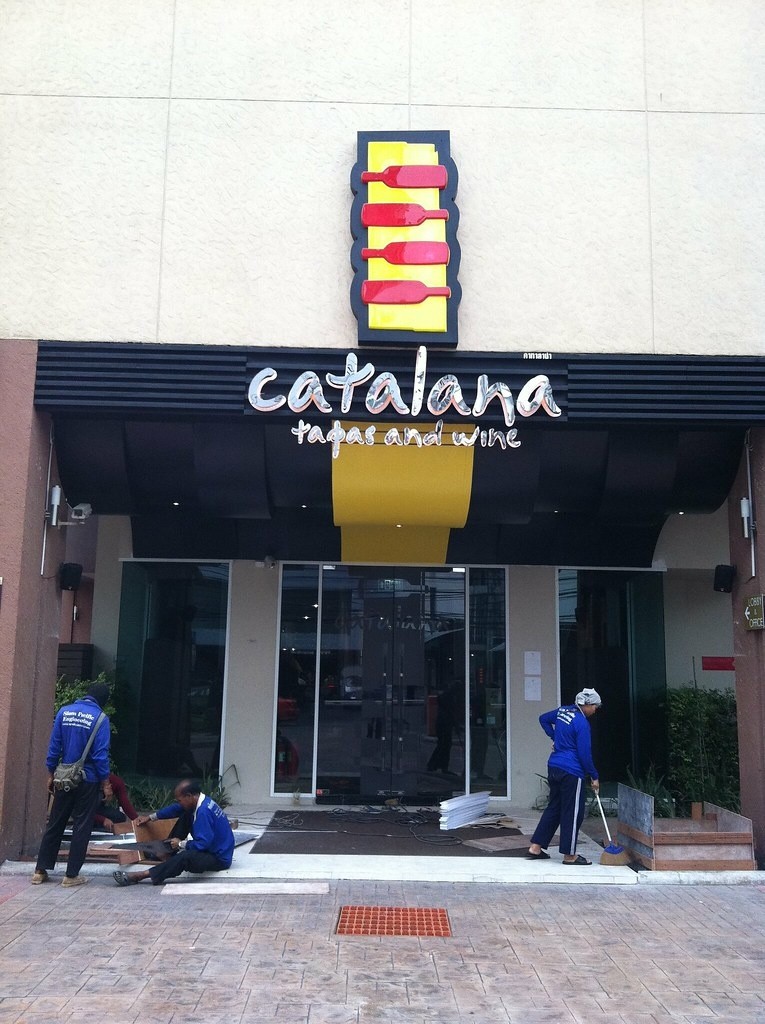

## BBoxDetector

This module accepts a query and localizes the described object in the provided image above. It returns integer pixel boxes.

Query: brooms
[588,772,636,867]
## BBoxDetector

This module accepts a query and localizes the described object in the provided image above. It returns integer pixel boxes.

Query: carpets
[337,906,452,937]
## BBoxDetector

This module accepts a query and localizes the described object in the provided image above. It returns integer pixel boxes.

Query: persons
[94,775,138,832]
[427,680,464,777]
[31,685,113,887]
[526,688,601,864]
[112,781,235,886]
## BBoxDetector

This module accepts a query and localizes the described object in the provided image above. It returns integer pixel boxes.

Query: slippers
[526,848,550,859]
[112,870,138,885]
[561,854,592,866]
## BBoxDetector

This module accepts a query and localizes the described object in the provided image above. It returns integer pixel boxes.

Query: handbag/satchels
[53,763,87,792]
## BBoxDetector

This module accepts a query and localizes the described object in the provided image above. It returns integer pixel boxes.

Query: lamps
[714,564,737,593]
[60,563,82,590]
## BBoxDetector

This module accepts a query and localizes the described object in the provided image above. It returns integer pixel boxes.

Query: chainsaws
[108,839,175,856]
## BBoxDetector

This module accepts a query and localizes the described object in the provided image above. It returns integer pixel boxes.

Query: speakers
[61,563,83,591]
[713,564,735,593]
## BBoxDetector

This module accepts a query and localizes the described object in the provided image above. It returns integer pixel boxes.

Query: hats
[81,683,109,708]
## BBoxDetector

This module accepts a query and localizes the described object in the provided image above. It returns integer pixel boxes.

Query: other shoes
[31,870,49,885]
[62,875,86,886]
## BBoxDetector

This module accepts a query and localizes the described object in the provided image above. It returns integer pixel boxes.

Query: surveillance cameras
[72,503,93,519]
[265,556,277,568]
